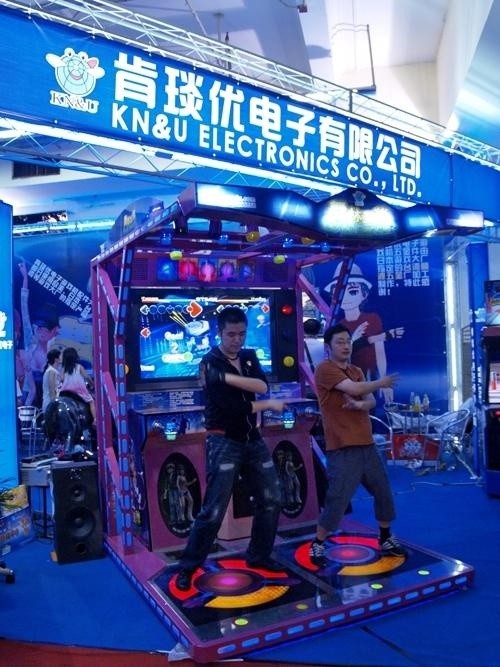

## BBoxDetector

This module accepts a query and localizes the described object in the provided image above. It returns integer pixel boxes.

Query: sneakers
[310,541,326,565]
[378,536,406,557]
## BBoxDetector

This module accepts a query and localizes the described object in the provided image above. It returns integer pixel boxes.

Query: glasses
[330,339,355,345]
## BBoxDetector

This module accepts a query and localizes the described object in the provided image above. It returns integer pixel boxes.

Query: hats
[324,261,372,292]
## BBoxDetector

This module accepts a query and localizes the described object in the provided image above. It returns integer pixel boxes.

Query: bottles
[409,392,430,413]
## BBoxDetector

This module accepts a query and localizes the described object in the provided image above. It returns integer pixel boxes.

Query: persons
[287,449,306,504]
[163,463,182,525]
[175,308,289,592]
[58,346,97,429]
[176,465,199,522]
[39,348,61,457]
[275,449,290,505]
[307,326,408,569]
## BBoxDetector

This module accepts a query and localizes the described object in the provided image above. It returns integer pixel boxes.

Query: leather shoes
[245,556,285,572]
[175,567,192,590]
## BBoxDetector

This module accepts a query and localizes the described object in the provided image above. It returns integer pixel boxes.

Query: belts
[205,428,226,436]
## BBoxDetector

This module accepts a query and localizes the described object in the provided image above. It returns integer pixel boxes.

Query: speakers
[50,461,103,565]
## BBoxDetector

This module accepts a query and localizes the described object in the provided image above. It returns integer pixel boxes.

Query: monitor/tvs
[131,289,277,382]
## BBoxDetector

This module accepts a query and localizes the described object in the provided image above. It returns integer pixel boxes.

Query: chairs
[381,403,479,482]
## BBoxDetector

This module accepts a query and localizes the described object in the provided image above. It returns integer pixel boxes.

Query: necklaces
[225,354,240,362]
